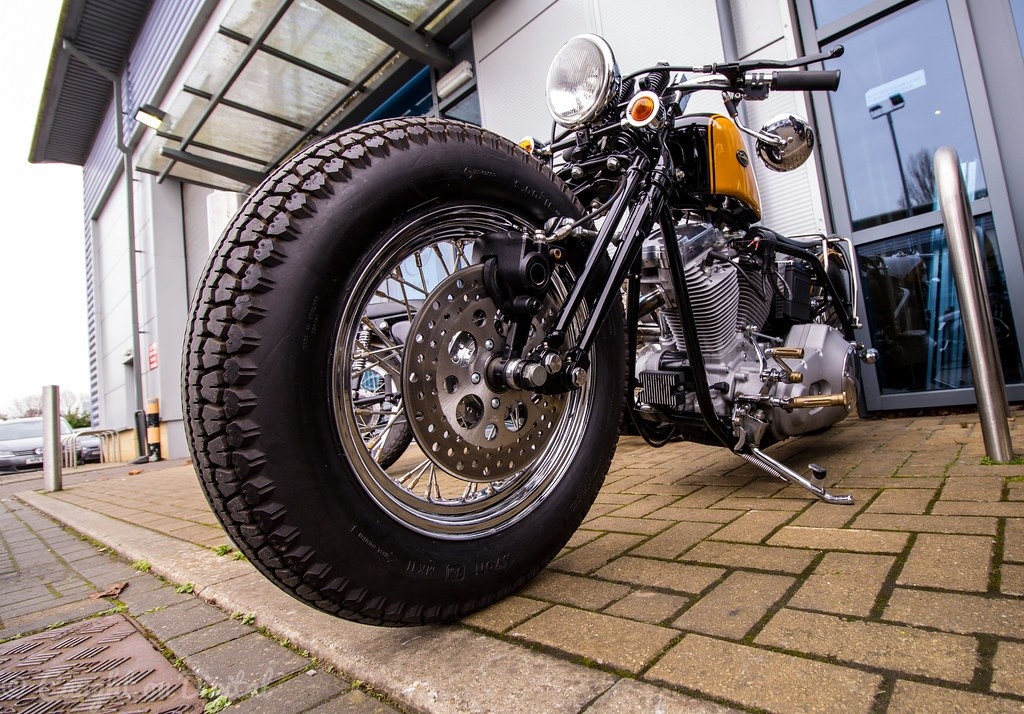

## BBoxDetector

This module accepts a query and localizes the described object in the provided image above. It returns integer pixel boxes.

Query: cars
[1,416,101,475]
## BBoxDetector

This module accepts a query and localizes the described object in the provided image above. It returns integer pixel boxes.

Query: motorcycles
[178,34,880,629]
